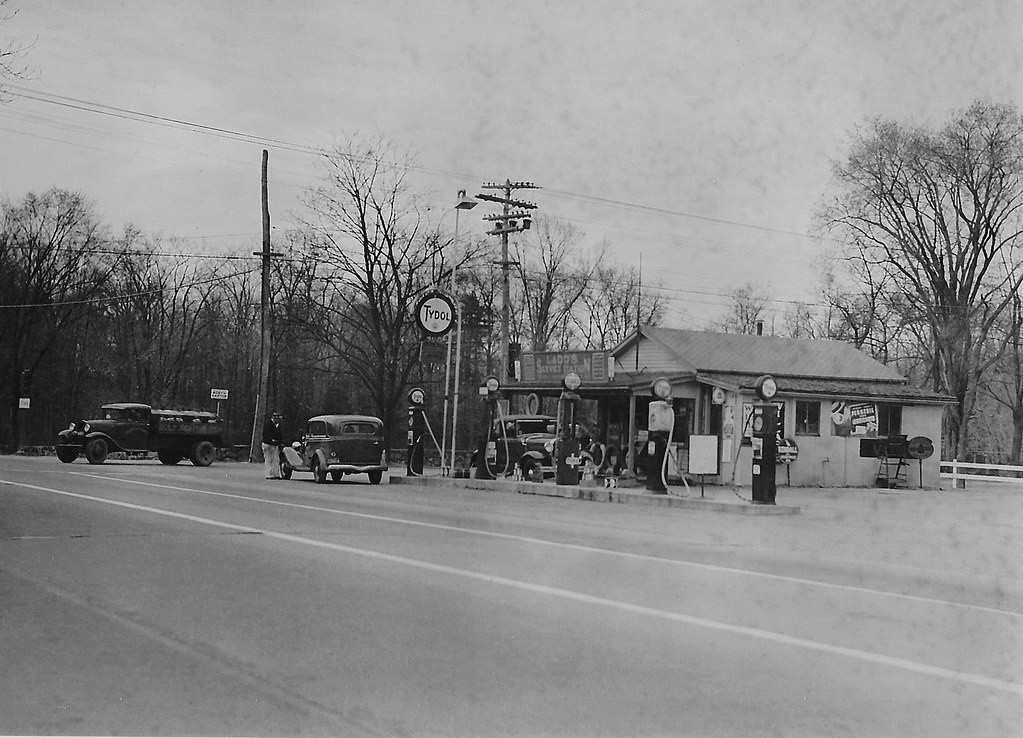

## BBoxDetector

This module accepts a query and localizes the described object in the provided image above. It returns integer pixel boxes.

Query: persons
[260,411,282,480]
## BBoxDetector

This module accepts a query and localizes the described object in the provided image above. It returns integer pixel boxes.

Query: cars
[488,413,596,481]
[279,415,388,484]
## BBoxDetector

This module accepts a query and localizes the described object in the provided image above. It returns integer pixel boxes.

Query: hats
[271,411,283,420]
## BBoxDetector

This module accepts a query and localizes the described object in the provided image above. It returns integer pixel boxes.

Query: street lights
[440,188,479,478]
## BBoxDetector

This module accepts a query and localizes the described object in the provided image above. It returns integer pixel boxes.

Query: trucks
[57,404,223,467]
